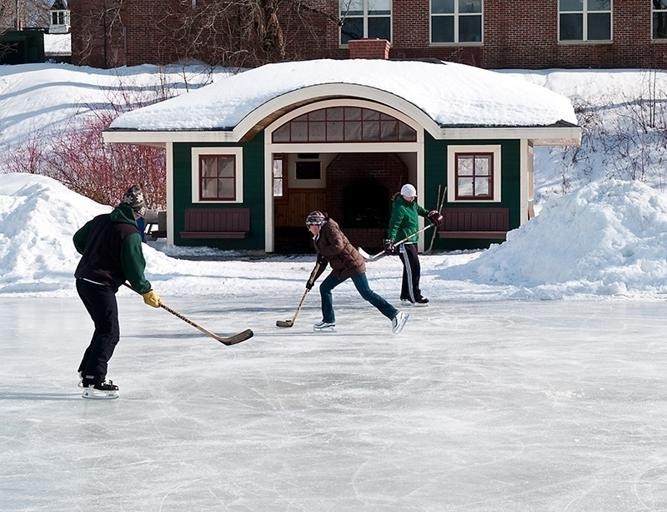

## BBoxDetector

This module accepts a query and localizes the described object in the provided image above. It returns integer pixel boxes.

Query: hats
[122,185,146,212]
[305,210,325,225]
[401,184,417,197]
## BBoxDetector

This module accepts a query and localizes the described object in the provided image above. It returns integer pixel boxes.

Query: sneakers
[81,371,119,390]
[313,321,335,329]
[392,311,405,334]
[400,296,429,303]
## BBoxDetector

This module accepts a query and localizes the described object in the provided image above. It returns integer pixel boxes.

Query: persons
[305,211,407,334]
[385,184,444,303]
[73,185,161,391]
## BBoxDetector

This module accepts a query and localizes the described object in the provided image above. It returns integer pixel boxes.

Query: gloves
[384,239,397,253]
[143,291,164,308]
[426,210,444,226]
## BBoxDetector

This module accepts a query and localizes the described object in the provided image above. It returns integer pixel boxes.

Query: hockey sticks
[276,288,309,327]
[123,282,253,346]
[358,215,443,261]
[417,184,448,254]
